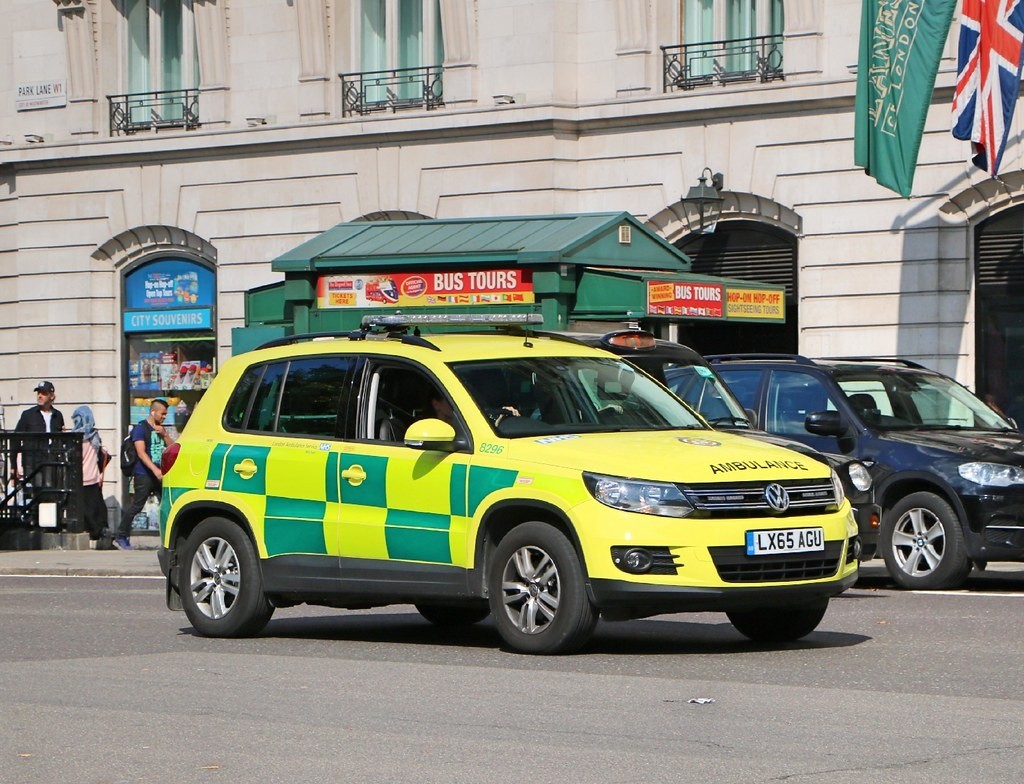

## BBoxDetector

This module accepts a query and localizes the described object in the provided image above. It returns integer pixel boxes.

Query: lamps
[681,166,725,204]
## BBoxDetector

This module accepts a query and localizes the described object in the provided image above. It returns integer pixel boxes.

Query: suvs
[697,353,1024,589]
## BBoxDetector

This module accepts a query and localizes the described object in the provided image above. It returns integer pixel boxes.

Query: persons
[406,373,520,449]
[70,406,109,549]
[113,400,175,549]
[11,380,66,527]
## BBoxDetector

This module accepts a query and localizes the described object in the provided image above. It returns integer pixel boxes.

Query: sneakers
[112,536,131,550]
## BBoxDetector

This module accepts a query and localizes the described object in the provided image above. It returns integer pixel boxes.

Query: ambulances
[159,308,861,655]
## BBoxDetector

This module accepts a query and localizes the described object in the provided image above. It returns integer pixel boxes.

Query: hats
[33,381,55,393]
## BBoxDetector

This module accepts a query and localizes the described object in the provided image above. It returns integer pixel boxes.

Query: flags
[951,0,1024,178]
[854,0,957,198]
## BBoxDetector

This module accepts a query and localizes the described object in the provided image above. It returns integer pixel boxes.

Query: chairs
[849,394,877,410]
[469,370,511,402]
[378,372,425,442]
[777,384,815,434]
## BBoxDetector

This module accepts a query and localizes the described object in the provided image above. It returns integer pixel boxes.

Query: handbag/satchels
[98,447,112,474]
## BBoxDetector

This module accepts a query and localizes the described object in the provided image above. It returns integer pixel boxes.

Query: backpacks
[120,420,151,487]
